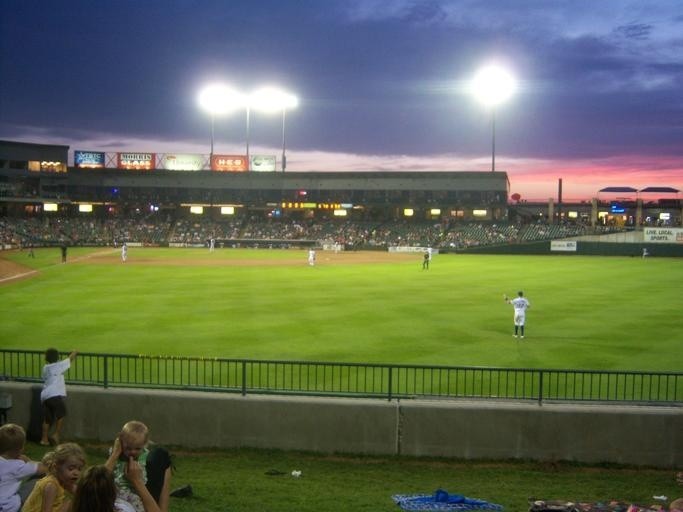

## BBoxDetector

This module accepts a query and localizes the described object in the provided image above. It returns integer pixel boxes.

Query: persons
[308,248,315,266]
[1,217,518,253]
[1,420,173,512]
[58,240,68,264]
[503,290,529,338]
[36,347,79,444]
[25,244,36,260]
[421,250,429,271]
[119,243,127,264]
[536,214,683,227]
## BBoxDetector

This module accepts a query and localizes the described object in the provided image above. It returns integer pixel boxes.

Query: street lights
[197,77,298,175]
[468,63,520,172]
